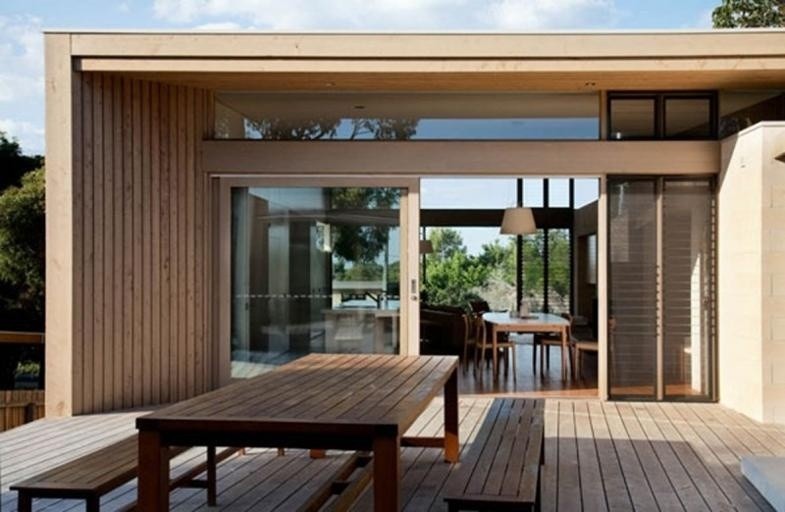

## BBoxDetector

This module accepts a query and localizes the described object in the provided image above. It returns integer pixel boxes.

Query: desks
[136,352,460,511]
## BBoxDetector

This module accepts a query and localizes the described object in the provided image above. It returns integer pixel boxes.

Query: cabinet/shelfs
[320,304,400,352]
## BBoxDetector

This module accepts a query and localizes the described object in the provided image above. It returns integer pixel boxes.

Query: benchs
[440,396,547,511]
[9,430,285,512]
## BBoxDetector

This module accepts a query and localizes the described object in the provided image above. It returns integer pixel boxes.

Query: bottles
[519,293,529,318]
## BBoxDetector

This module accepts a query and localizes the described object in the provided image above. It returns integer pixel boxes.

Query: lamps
[419,238,434,285]
[500,206,539,313]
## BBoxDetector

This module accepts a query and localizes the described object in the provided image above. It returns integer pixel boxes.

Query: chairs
[420,299,618,385]
